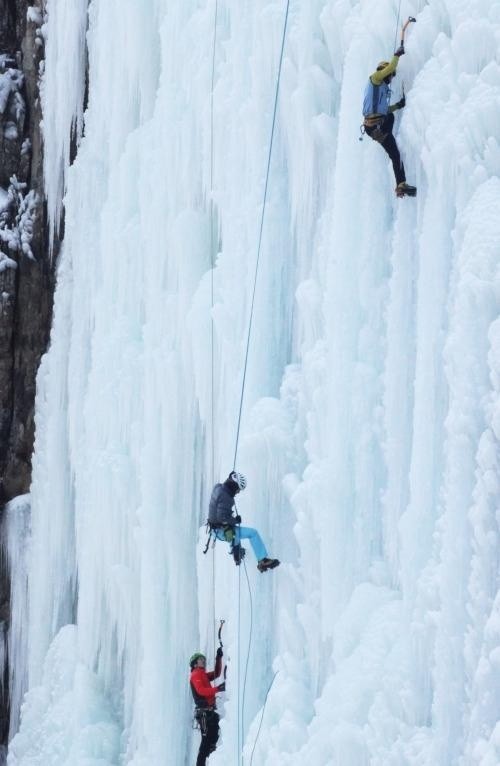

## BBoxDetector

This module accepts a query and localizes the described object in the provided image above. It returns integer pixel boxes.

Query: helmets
[231,472,247,490]
[189,652,206,667]
[377,61,396,77]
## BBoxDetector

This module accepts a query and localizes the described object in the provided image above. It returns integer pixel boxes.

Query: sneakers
[257,557,279,573]
[234,547,245,565]
[395,183,416,198]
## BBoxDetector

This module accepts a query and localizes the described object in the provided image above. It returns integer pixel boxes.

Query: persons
[204,469,281,573]
[360,48,419,198]
[187,646,228,766]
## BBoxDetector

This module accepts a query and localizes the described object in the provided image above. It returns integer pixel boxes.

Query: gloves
[393,47,404,57]
[217,647,223,656]
[395,98,405,110]
[218,682,225,692]
[236,515,241,524]
